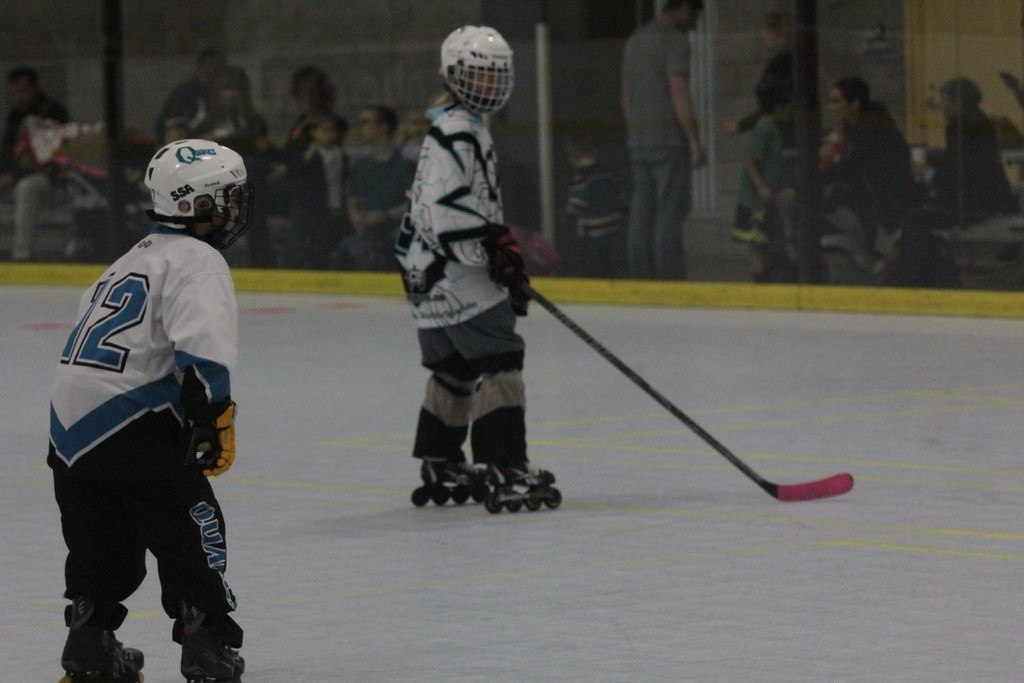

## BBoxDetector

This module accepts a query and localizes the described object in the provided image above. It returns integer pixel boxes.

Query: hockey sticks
[521,280,855,502]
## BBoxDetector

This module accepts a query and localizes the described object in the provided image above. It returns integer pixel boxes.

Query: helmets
[145,138,253,252]
[440,26,515,115]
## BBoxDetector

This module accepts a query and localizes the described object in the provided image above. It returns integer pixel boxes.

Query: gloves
[509,276,530,318]
[192,402,239,477]
[481,227,526,284]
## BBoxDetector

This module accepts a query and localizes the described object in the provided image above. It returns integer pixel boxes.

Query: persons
[392,26,561,514]
[0,14,1024,291]
[620,1,704,280]
[47,138,253,682]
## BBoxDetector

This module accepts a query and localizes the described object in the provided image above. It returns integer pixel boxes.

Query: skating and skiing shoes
[180,607,245,682]
[60,603,144,682]
[474,460,562,514]
[409,458,488,507]
